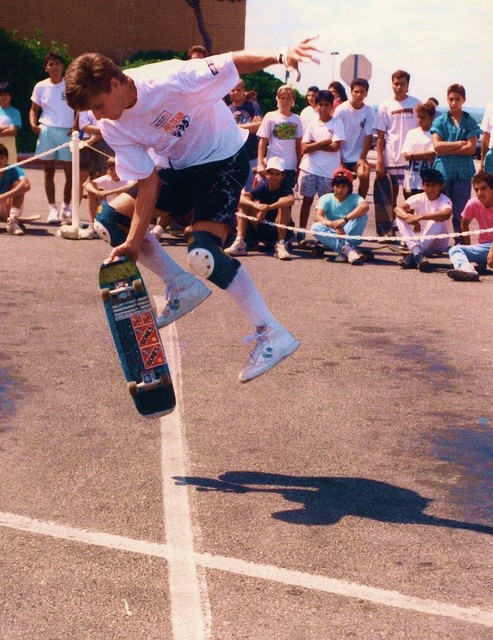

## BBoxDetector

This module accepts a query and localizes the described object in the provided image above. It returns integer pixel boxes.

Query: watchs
[343,216,349,223]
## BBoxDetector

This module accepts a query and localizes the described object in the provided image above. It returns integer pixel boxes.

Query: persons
[479,100,493,174]
[393,169,456,273]
[299,86,319,130]
[63,33,325,383]
[446,170,492,282]
[244,90,258,102]
[223,156,295,260]
[297,90,347,249]
[60,109,115,225]
[227,78,263,194]
[0,79,22,166]
[147,208,195,241]
[78,157,138,239]
[255,84,303,188]
[370,70,422,243]
[328,82,348,117]
[332,78,376,200]
[429,84,483,246]
[0,143,31,236]
[400,97,437,201]
[29,52,79,225]
[311,167,369,264]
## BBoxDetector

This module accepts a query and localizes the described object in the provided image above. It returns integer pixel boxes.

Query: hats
[331,168,353,182]
[419,169,443,181]
[265,157,285,171]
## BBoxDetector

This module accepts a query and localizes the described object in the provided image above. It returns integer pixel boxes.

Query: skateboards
[449,259,488,270]
[249,232,305,251]
[0,214,41,230]
[158,232,185,245]
[372,174,394,237]
[97,257,176,420]
[386,243,452,259]
[306,241,388,262]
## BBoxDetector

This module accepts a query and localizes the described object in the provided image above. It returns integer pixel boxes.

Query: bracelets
[277,45,289,67]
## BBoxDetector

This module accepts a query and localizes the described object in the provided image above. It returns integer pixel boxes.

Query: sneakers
[59,210,71,219]
[418,257,431,271]
[342,245,365,263]
[238,323,300,382]
[274,243,291,258]
[334,254,347,261]
[225,240,248,255]
[48,208,59,223]
[398,255,416,267]
[447,265,479,280]
[154,272,211,329]
[6,218,23,234]
